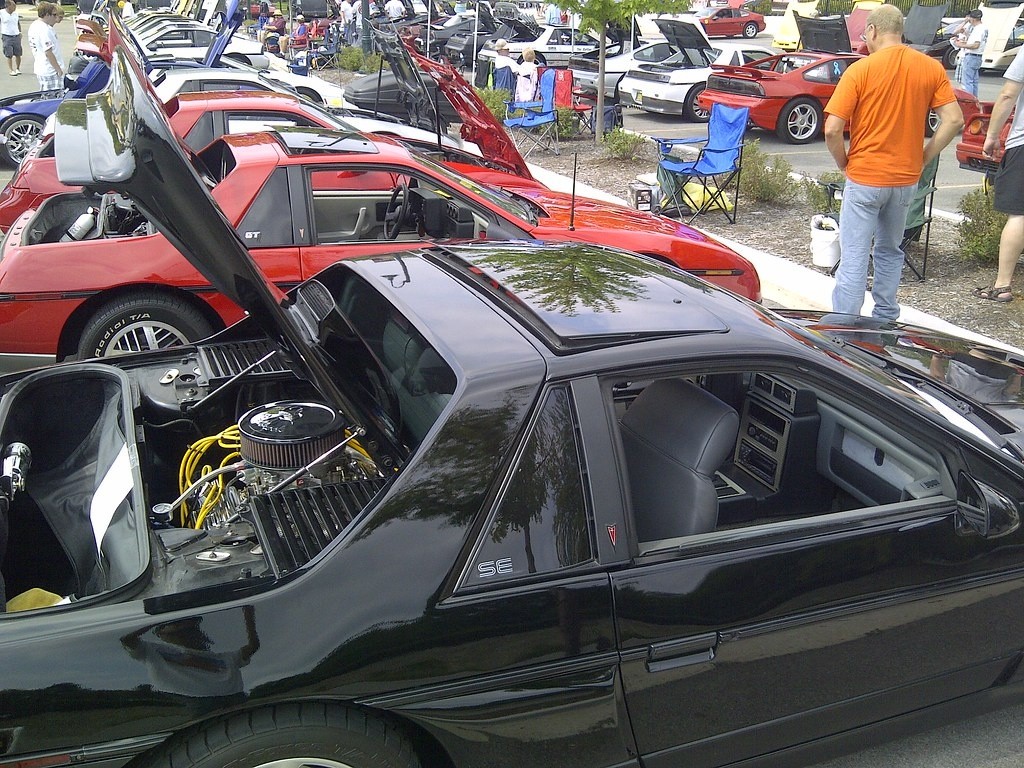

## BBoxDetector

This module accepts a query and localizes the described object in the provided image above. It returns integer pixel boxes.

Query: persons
[341,0,375,48]
[972,44,1024,302]
[954,9,988,97]
[257,9,286,51]
[518,47,546,75]
[823,3,964,322]
[545,4,571,26]
[0,0,23,76]
[276,15,306,58]
[122,0,134,19]
[28,1,65,91]
[494,39,521,72]
[384,0,406,23]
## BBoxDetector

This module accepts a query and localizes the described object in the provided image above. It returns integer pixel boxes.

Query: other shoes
[16,69,22,74]
[9,71,17,76]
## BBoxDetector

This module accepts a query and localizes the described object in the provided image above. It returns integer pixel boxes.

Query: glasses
[52,14,56,16]
[857,23,872,41]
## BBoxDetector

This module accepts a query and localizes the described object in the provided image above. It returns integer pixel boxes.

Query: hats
[273,9,283,16]
[293,15,305,21]
[966,9,983,20]
[495,39,510,51]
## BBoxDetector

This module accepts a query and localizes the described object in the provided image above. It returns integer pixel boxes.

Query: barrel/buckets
[811,214,840,268]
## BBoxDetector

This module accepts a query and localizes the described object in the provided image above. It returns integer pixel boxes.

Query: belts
[965,53,982,57]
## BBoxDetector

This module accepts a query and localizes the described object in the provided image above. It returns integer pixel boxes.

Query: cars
[771,0,1024,72]
[0,40,1024,767]
[698,50,979,144]
[1,0,764,374]
[956,99,1016,180]
[618,10,852,123]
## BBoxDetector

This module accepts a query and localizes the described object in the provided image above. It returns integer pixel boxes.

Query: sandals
[972,281,1013,302]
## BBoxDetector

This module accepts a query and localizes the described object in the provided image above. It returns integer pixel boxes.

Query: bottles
[59,211,98,241]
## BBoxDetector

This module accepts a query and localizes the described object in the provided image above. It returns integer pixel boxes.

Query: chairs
[816,152,939,279]
[651,102,749,225]
[383,321,448,444]
[474,57,597,160]
[247,13,359,78]
[618,378,740,540]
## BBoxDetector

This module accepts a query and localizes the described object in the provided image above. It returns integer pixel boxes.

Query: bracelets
[19,32,22,34]
